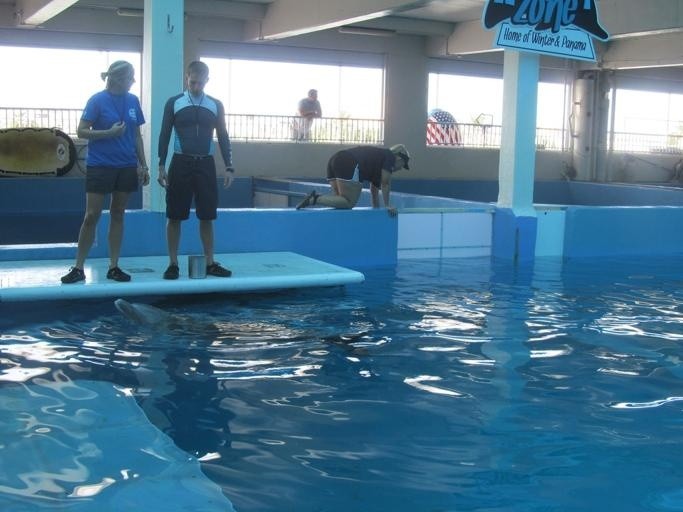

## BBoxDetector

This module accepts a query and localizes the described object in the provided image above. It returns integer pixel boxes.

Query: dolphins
[114,299,366,344]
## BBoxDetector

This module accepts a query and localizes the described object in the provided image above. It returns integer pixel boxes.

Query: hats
[398,152,409,170]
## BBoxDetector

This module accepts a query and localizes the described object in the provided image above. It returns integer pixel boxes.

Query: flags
[427,109,462,146]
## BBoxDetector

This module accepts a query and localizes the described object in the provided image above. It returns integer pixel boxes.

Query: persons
[291,89,322,140]
[157,60,235,279]
[60,60,149,283]
[296,144,411,217]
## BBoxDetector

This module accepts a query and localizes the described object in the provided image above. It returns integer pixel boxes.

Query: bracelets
[140,166,148,170]
[226,168,234,173]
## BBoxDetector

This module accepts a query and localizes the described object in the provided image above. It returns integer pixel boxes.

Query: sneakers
[107,267,130,281]
[206,263,231,277]
[163,263,179,279]
[61,267,85,283]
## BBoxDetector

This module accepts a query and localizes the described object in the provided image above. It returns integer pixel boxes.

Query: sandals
[295,191,318,209]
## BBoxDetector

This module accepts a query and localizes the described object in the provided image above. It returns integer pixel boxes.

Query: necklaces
[189,91,204,137]
[110,92,126,124]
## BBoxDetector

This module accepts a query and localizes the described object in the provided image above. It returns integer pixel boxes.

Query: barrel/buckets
[187,252,210,281]
